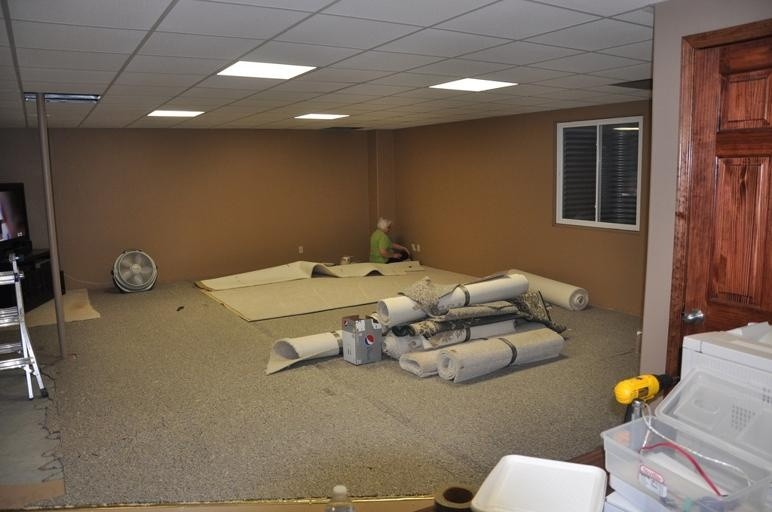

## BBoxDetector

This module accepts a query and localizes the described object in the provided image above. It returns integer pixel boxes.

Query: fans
[109,247,160,293]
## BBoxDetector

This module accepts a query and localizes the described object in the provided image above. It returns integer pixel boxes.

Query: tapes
[437,484,477,510]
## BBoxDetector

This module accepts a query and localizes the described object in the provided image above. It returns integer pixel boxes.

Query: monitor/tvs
[0,183,32,259]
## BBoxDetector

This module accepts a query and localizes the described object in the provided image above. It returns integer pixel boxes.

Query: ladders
[0,254,49,400]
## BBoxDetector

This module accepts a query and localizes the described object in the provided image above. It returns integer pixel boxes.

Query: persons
[369,218,410,264]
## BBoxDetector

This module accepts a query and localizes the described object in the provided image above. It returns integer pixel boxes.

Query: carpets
[0,288,101,331]
[29,260,643,511]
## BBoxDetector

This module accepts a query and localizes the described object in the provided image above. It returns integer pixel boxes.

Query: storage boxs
[340,312,383,366]
[599,320,772,512]
[470,453,608,511]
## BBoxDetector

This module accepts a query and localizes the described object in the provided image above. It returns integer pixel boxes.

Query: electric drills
[614,374,680,424]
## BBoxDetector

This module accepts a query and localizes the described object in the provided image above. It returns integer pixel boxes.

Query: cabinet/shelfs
[0,247,56,316]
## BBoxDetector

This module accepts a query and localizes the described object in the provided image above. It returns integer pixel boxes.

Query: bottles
[325,485,355,512]
